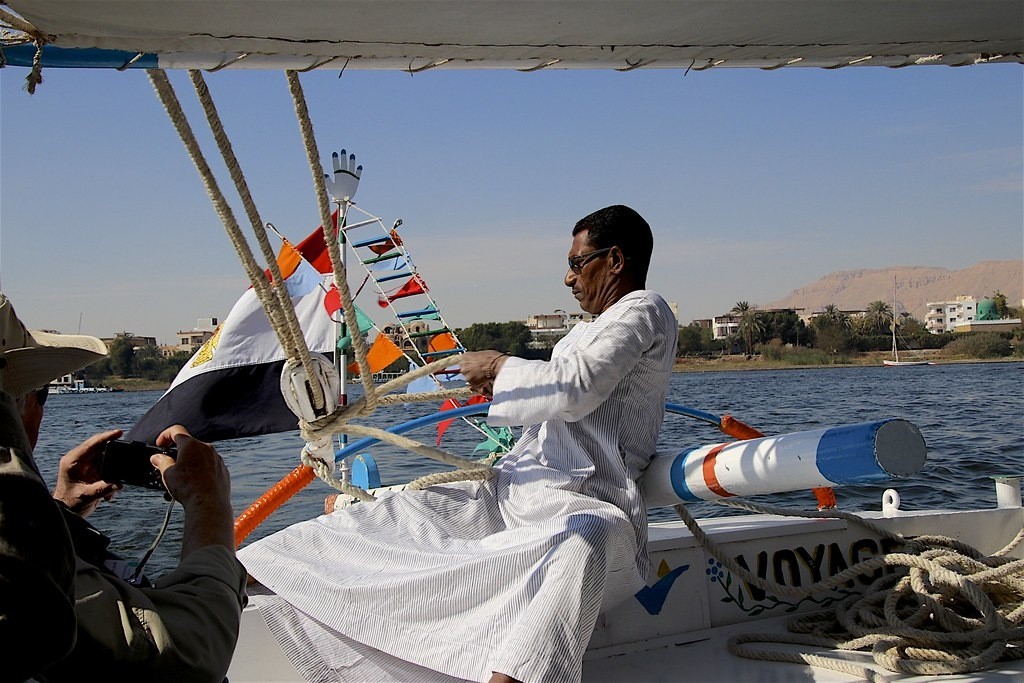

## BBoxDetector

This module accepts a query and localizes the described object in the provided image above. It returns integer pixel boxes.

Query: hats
[0,293,109,398]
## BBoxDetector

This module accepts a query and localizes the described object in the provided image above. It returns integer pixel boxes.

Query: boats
[352,372,403,384]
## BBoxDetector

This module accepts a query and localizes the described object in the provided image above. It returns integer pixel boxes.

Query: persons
[235,204,679,683]
[0,292,248,683]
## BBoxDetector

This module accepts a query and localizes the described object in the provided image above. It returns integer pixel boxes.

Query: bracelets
[485,353,507,379]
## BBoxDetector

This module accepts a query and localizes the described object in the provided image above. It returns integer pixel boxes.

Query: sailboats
[883,273,928,366]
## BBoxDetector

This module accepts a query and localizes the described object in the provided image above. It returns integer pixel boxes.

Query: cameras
[100,438,179,492]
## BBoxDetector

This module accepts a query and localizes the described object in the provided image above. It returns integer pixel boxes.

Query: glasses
[30,384,52,405]
[569,247,630,276]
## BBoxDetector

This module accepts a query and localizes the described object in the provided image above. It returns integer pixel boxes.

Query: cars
[696,351,713,356]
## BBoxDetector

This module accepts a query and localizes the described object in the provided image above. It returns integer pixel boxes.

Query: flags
[294,209,339,275]
[123,237,336,448]
